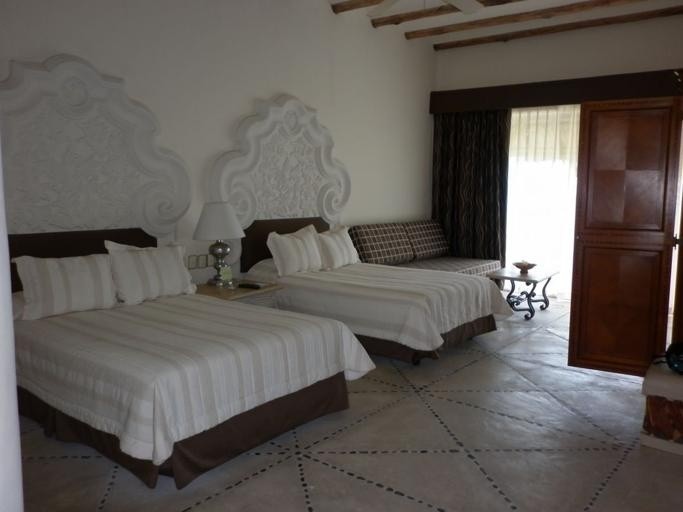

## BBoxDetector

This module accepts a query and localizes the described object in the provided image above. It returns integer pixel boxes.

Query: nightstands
[196,280,283,308]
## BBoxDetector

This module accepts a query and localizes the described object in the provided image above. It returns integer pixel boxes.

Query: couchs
[386,256,502,289]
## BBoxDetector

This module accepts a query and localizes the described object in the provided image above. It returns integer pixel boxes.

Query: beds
[241,217,514,366]
[8,228,377,489]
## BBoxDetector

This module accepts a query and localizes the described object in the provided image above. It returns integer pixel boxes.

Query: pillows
[314,226,361,272]
[10,254,118,320]
[104,241,198,306]
[348,223,415,265]
[266,224,324,277]
[401,220,449,262]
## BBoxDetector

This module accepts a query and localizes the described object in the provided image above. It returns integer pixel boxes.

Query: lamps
[207,240,231,286]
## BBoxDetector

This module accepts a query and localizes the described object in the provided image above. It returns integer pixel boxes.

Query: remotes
[238,282,264,290]
